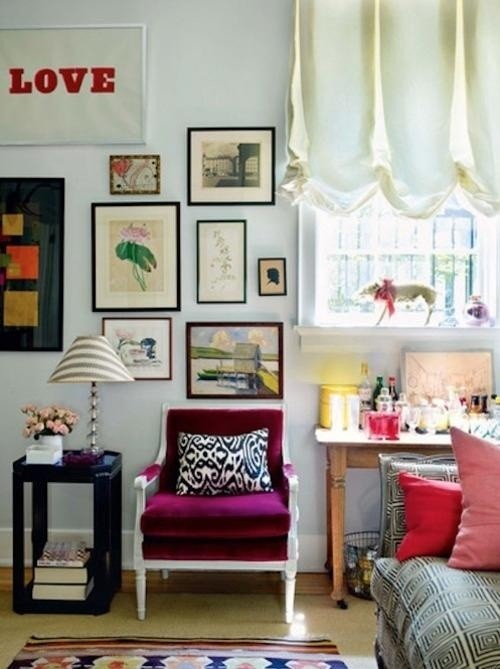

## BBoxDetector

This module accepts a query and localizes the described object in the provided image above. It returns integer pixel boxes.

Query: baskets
[344,529,379,600]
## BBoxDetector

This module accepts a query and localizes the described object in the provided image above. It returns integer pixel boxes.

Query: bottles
[358,361,499,440]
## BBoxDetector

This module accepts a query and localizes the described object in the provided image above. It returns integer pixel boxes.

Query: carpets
[7,633,348,669]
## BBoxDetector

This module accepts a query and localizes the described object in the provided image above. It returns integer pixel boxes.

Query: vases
[38,435,64,460]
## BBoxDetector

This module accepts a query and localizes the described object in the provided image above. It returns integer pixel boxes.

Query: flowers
[22,405,81,441]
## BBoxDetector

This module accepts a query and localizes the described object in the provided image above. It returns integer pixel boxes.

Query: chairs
[130,403,301,628]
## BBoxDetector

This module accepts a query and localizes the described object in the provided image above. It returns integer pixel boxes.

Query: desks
[11,449,123,615]
[312,421,456,609]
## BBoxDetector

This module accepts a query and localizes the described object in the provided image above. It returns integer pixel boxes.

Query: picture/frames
[397,346,498,407]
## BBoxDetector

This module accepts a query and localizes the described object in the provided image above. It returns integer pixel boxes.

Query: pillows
[377,426,500,571]
[173,426,274,497]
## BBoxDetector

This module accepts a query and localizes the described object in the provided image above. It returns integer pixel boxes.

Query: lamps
[44,335,136,453]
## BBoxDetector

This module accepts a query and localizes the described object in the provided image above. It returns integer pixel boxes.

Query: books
[30,539,96,603]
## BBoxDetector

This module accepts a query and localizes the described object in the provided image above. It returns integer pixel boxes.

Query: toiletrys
[330,389,500,436]
